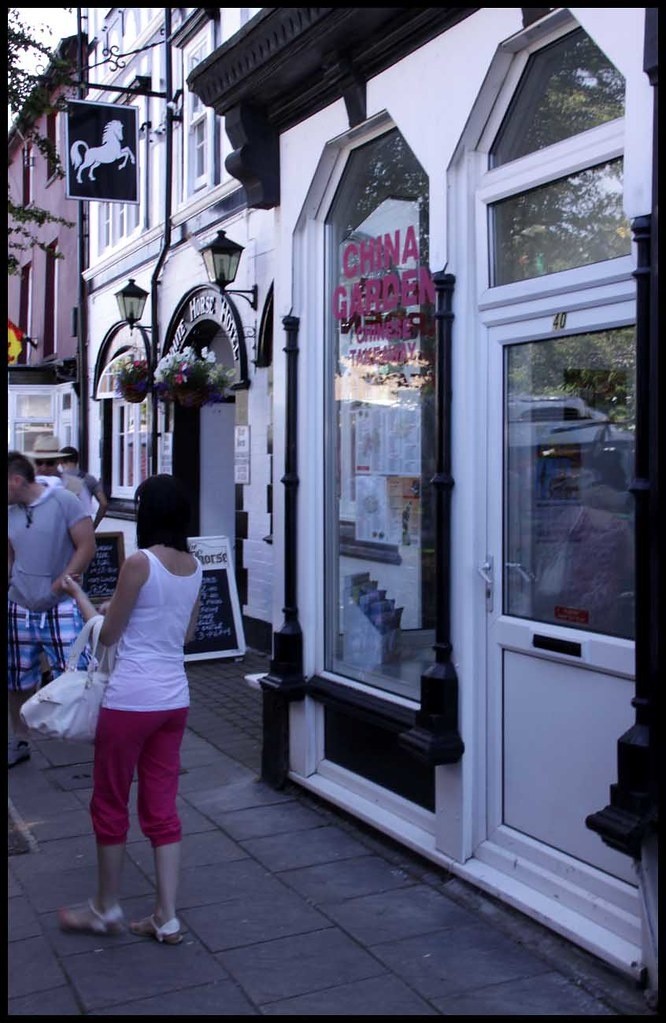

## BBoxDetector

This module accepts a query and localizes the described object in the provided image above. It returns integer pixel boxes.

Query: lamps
[113,278,152,334]
[151,343,239,403]
[197,228,258,312]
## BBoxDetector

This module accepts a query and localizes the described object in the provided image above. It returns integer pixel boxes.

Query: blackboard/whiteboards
[79,531,126,603]
[183,568,246,662]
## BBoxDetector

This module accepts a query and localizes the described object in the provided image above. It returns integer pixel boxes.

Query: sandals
[58,898,124,934]
[129,913,184,943]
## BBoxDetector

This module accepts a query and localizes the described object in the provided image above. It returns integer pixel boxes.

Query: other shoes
[8,740,31,766]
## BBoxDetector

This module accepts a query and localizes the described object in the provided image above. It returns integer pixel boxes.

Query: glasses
[35,458,57,466]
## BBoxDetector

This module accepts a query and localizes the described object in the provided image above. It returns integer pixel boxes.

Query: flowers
[110,357,150,399]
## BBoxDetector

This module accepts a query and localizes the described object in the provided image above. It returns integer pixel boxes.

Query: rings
[66,578,72,581]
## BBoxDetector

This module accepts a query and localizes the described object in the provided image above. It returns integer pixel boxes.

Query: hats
[22,435,72,458]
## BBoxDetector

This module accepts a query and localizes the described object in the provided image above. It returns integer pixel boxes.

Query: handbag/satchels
[539,506,582,595]
[20,615,117,744]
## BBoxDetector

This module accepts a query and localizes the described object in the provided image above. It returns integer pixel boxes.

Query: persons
[61,476,206,944]
[25,438,92,520]
[8,450,96,764]
[61,446,107,531]
[541,465,635,634]
